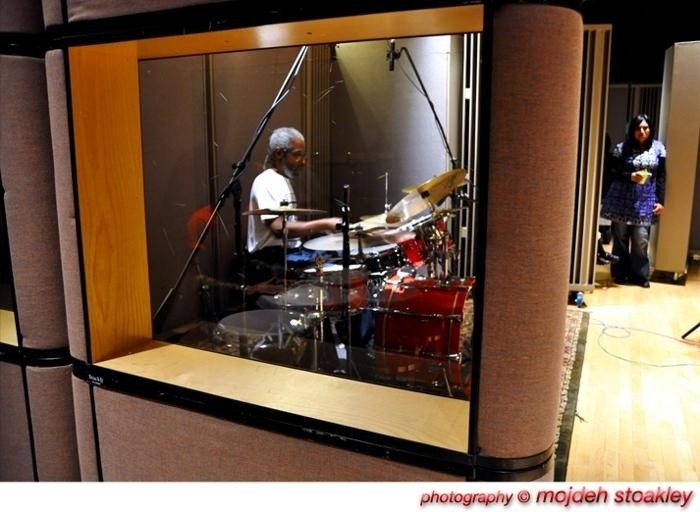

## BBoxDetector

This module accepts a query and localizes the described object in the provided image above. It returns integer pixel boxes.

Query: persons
[187,172,281,305]
[596,228,621,266]
[241,124,378,375]
[599,130,617,245]
[602,111,668,289]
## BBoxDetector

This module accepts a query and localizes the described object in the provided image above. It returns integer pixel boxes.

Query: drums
[305,264,367,318]
[369,274,475,400]
[258,283,364,370]
[213,309,311,367]
[368,215,456,270]
[339,243,404,273]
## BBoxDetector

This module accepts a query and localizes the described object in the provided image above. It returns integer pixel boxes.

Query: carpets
[458,297,589,482]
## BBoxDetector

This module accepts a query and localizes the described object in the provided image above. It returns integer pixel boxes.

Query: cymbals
[242,208,327,216]
[303,226,416,251]
[386,169,466,223]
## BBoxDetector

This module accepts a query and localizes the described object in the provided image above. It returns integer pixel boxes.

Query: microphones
[389,38,396,72]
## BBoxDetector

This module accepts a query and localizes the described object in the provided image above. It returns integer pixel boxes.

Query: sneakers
[597,252,619,265]
